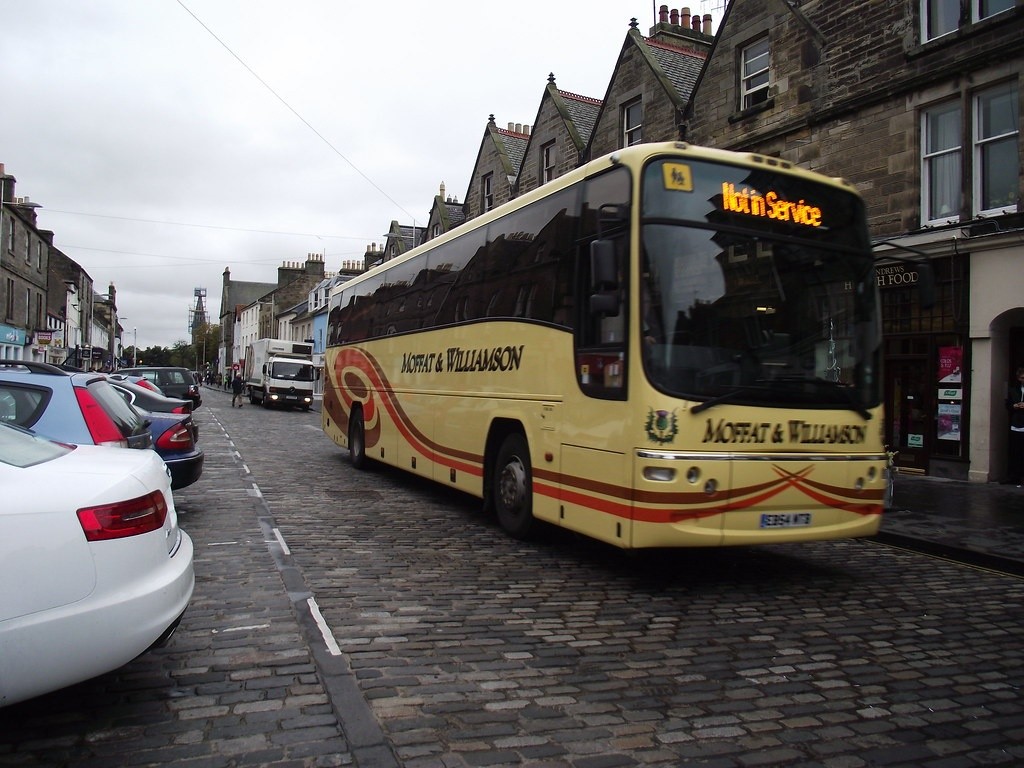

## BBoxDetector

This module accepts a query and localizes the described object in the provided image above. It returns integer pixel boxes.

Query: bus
[321,140,939,551]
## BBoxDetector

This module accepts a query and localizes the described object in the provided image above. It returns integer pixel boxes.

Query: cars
[131,405,203,491]
[106,376,199,443]
[0,420,196,708]
[111,367,202,411]
[126,376,166,397]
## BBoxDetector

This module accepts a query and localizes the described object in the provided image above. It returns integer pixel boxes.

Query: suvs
[0,359,155,451]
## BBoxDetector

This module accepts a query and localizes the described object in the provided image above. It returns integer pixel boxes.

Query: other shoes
[239,404,242,407]
[232,401,235,407]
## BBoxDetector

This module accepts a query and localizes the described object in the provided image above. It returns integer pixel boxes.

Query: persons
[194,371,246,394]
[232,372,243,408]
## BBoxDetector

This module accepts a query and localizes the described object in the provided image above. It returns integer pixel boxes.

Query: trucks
[244,338,320,411]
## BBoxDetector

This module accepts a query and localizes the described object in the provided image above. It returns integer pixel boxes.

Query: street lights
[90,292,113,368]
[0,180,43,238]
[112,316,127,367]
[383,227,415,249]
[256,294,275,339]
